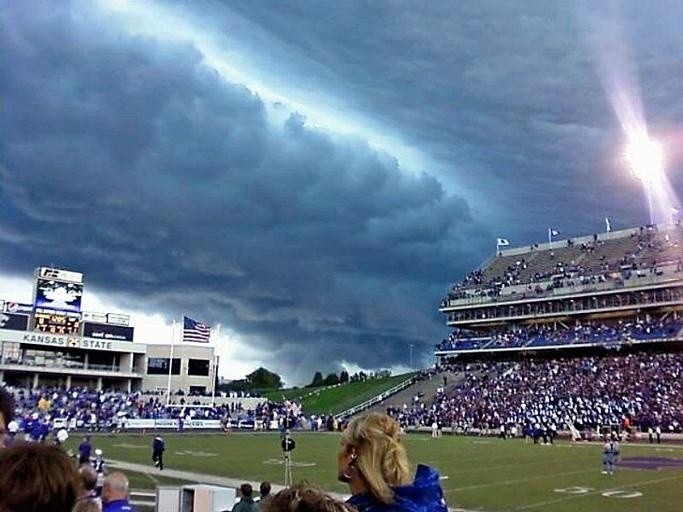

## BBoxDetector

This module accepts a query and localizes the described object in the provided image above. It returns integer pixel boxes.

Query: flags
[183,315,211,343]
